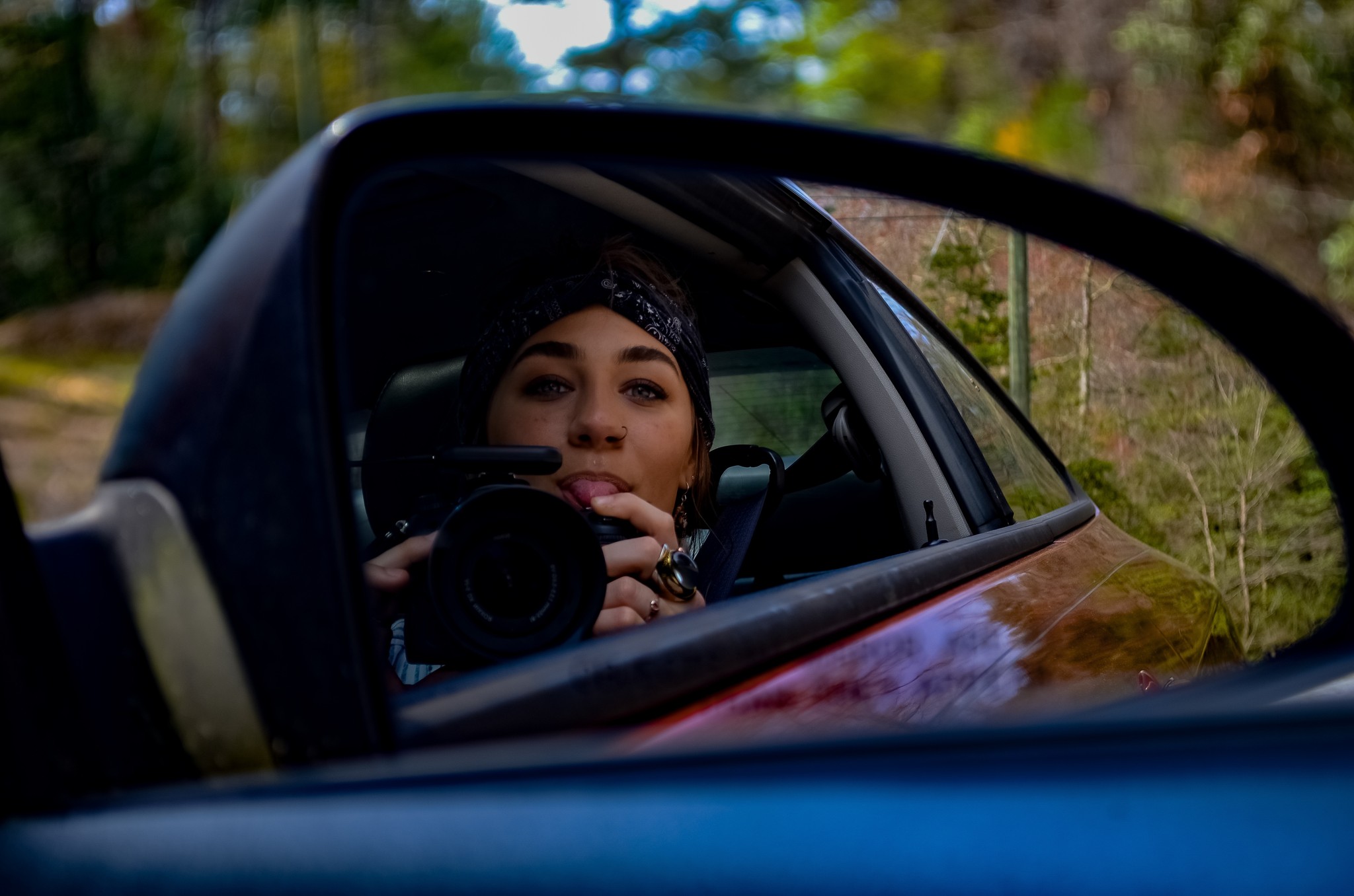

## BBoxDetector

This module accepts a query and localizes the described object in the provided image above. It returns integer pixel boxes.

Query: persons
[364,235,715,690]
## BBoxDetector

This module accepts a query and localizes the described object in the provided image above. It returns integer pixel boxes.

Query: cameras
[374,447,650,675]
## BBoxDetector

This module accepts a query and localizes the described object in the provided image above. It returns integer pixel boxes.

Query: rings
[652,546,699,603]
[644,595,660,623]
